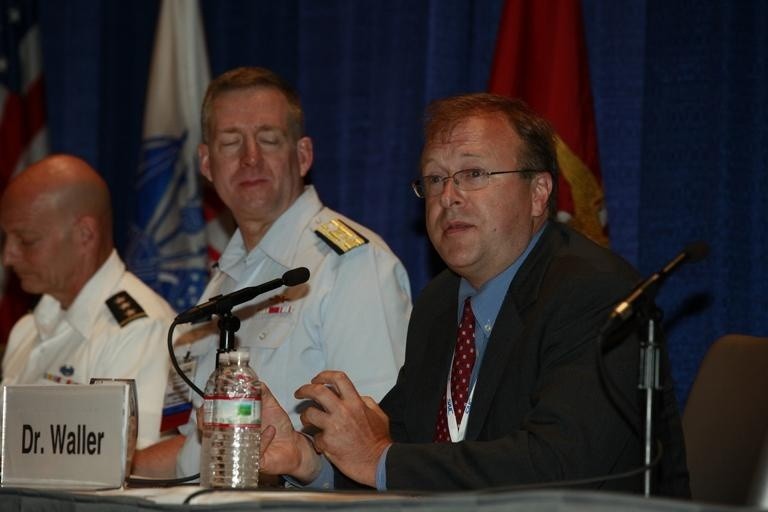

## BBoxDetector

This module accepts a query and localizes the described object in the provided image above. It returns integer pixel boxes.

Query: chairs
[679,333,768,507]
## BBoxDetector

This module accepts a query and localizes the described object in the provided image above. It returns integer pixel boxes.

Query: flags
[0,0,57,378]
[125,0,237,330]
[491,2,619,254]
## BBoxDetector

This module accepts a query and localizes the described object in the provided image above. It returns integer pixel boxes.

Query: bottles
[213,350,263,489]
[199,352,232,489]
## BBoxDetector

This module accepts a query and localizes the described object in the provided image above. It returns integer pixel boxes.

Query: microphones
[603,241,709,332]
[175,267,310,325]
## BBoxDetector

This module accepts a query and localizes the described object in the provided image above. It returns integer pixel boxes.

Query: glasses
[411,167,547,199]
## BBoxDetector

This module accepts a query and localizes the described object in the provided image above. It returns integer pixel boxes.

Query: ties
[433,296,478,443]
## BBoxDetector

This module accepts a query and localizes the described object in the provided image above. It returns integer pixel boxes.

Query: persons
[160,65,414,480]
[0,155,188,484]
[208,93,691,499]
